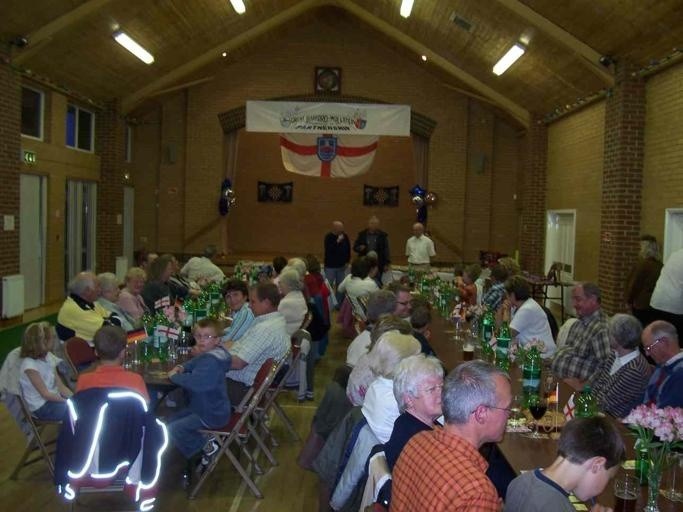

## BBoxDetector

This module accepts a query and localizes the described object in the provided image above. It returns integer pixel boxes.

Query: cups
[665,450,683,502]
[130,337,141,364]
[122,342,130,368]
[510,394,523,429]
[612,474,639,512]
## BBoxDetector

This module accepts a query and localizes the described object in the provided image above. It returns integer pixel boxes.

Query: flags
[280,133,378,179]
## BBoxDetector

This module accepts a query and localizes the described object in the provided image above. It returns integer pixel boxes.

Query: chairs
[1,260,683,512]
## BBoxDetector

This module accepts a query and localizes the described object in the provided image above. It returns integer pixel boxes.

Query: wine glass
[527,390,546,438]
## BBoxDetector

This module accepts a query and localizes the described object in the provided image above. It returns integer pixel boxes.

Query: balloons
[219,178,239,216]
[409,184,436,224]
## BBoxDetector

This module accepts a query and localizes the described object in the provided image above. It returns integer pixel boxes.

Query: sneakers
[201,440,220,466]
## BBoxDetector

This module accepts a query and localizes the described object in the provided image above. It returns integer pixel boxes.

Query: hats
[465,264,481,282]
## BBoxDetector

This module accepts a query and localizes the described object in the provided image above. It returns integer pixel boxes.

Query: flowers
[620,403,682,511]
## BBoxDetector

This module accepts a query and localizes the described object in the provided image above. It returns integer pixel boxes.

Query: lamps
[110,27,155,66]
[491,42,527,77]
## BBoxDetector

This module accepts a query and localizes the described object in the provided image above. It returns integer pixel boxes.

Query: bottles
[573,385,597,418]
[408,264,510,370]
[522,345,542,393]
[141,260,275,364]
[634,432,649,483]
[549,263,563,281]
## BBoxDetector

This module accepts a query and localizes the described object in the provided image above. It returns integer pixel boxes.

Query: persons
[650,249,683,347]
[58,254,223,373]
[545,282,610,392]
[219,255,325,405]
[390,361,512,512]
[353,215,391,287]
[385,353,444,471]
[505,415,626,512]
[405,222,437,272]
[322,221,351,309]
[587,314,651,420]
[642,321,683,409]
[166,317,231,466]
[455,258,559,358]
[626,236,663,323]
[75,326,149,403]
[338,258,445,445]
[20,322,73,421]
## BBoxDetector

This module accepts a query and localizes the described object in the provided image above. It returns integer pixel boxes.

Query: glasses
[471,404,512,419]
[643,339,662,353]
